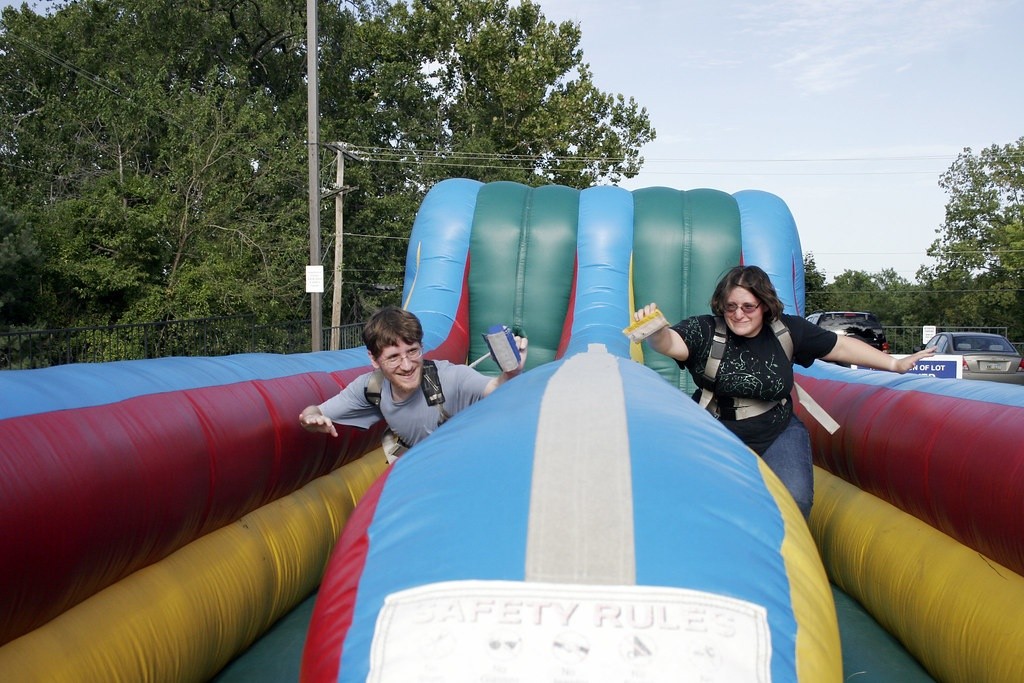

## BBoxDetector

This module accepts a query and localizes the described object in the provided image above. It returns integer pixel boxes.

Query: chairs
[957,344,971,350]
[989,345,1003,350]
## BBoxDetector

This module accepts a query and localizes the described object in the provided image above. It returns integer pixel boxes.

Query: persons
[634,266,938,522]
[299,306,528,450]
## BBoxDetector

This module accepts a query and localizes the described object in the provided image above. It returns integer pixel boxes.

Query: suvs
[803,309,889,368]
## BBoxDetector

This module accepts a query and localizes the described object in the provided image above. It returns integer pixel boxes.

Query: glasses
[721,300,763,312]
[379,346,425,368]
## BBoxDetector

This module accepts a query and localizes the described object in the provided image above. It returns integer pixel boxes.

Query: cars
[919,331,1024,386]
